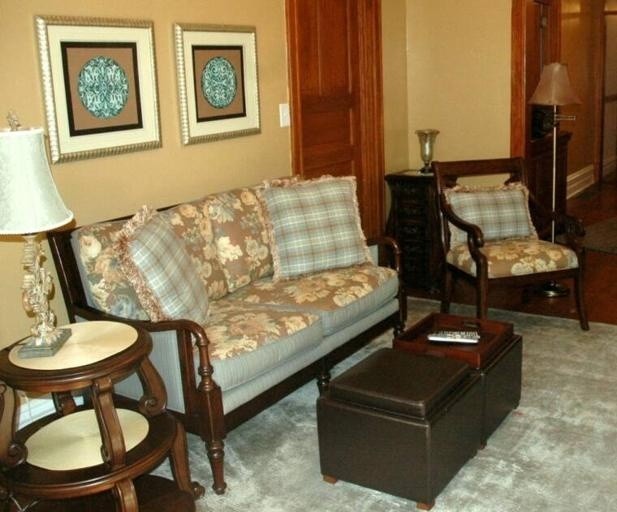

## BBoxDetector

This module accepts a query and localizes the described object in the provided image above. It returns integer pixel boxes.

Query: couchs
[47,173,404,495]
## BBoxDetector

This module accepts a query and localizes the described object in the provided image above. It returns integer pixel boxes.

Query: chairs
[431,157,589,332]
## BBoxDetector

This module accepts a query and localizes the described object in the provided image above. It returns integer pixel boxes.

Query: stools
[0,319,204,508]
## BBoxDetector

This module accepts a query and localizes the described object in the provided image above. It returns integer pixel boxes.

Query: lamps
[0,127,74,359]
[525,58,583,299]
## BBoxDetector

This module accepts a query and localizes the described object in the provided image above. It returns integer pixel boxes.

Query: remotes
[426,330,478,344]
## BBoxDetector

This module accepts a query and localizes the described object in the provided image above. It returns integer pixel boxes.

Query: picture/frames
[171,22,263,147]
[33,15,163,165]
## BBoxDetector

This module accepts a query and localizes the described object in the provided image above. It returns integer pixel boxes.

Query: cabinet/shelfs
[525,129,572,243]
[384,167,459,292]
